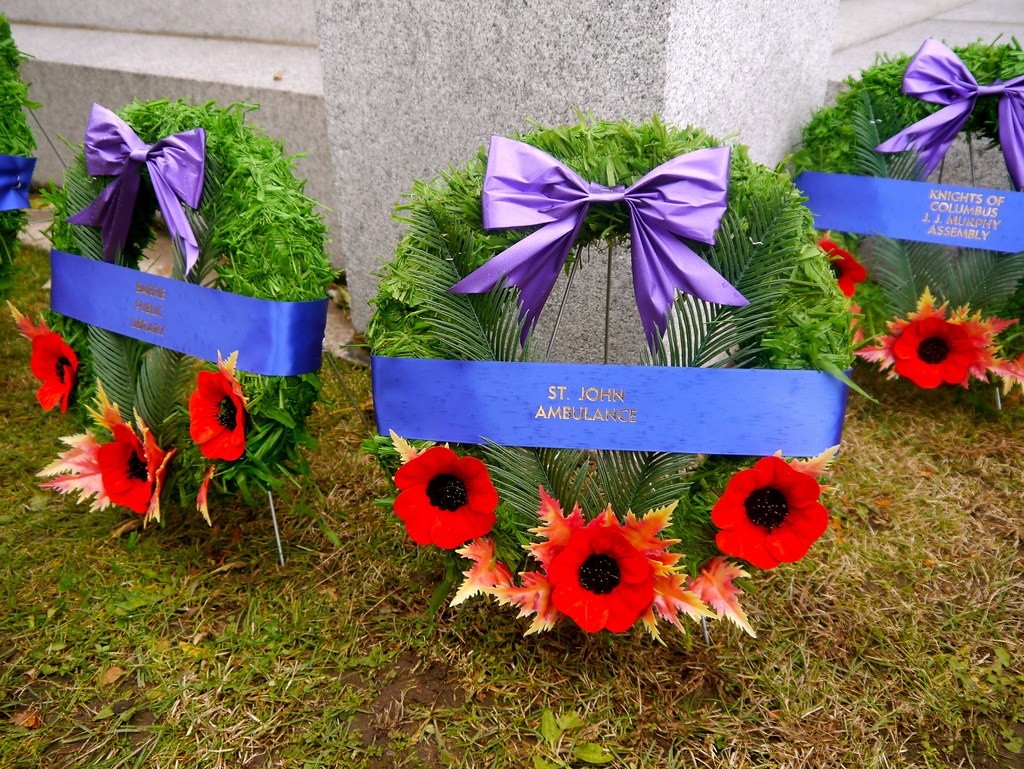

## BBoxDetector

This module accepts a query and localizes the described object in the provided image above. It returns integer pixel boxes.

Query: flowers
[4,99,347,529]
[788,32,1024,411]
[353,110,880,649]
